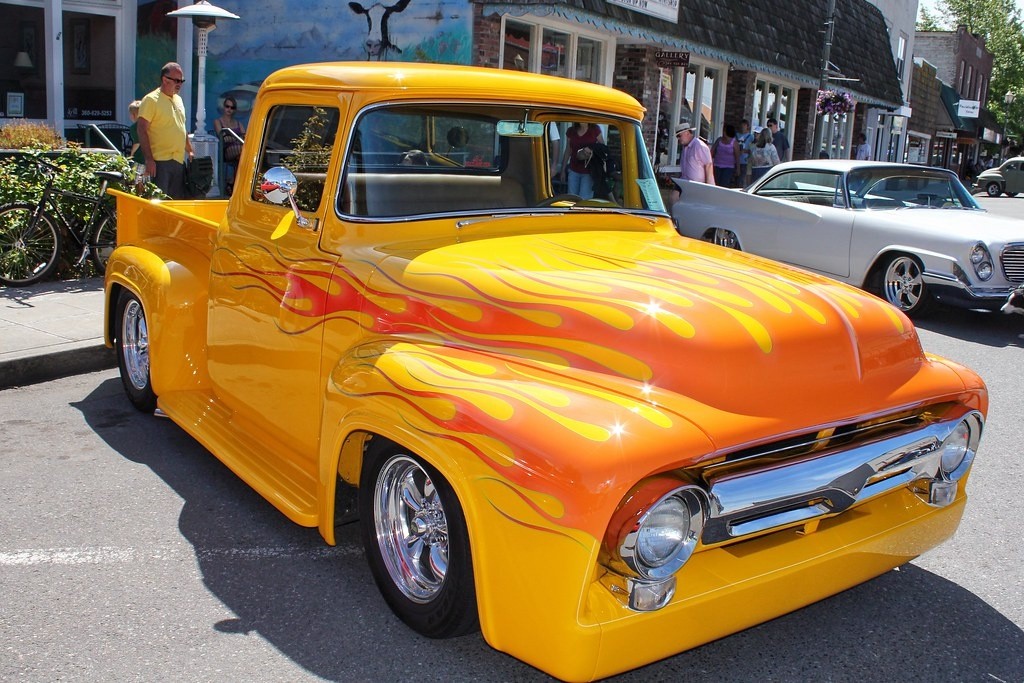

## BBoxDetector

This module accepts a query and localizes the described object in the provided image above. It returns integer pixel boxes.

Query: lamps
[13,53,34,68]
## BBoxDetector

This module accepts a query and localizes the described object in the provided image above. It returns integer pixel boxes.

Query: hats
[674,123,697,137]
[753,126,763,134]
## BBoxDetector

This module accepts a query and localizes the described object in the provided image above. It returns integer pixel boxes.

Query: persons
[513,121,560,177]
[672,123,716,186]
[818,153,829,159]
[137,62,194,200]
[214,97,245,199]
[403,150,426,166]
[710,125,740,189]
[855,133,871,160]
[736,119,791,187]
[968,155,993,181]
[128,100,145,174]
[560,122,603,201]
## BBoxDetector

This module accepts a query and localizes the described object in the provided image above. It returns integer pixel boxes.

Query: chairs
[77,122,134,157]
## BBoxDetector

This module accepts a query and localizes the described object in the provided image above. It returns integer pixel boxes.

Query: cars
[972,155,1024,196]
[659,160,1024,325]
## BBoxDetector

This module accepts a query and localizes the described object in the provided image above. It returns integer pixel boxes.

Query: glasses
[165,76,185,84]
[767,124,775,127]
[224,104,236,110]
[676,132,689,138]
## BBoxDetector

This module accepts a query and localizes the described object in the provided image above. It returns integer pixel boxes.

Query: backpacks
[734,132,751,151]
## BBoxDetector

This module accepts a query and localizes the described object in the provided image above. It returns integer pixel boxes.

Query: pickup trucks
[98,59,986,683]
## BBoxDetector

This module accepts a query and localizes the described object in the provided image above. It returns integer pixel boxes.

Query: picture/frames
[19,22,38,75]
[67,18,90,74]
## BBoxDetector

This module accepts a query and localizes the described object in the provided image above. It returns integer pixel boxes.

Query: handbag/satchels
[711,136,721,159]
[182,155,213,198]
[577,148,592,167]
[222,134,242,159]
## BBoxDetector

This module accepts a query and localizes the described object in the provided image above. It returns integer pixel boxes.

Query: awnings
[936,84,976,132]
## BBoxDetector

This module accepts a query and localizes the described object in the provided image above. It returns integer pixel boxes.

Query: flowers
[815,88,855,119]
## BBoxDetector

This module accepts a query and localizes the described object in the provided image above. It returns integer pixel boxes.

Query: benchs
[773,193,866,209]
[209,130,237,182]
[292,171,526,217]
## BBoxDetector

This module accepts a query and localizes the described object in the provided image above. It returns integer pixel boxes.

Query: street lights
[166,0,251,214]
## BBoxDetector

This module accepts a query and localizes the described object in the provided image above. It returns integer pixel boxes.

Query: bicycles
[0,148,173,287]
